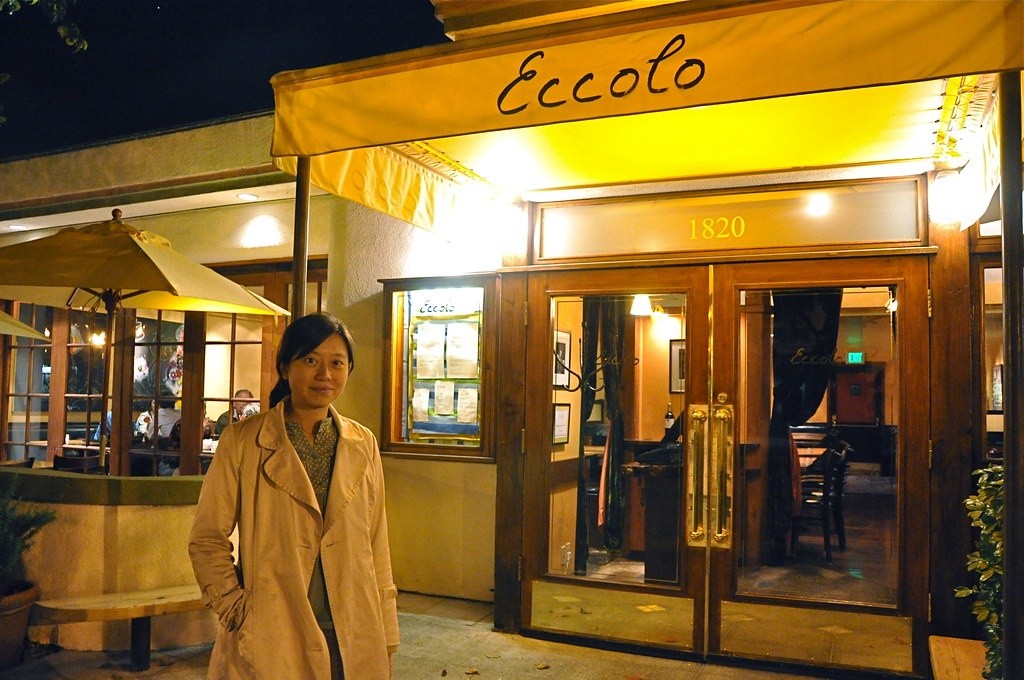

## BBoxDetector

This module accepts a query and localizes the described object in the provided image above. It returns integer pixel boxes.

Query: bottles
[665,403,675,434]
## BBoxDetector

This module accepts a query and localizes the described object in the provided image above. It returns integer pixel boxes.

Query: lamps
[628,295,653,316]
[65,293,145,355]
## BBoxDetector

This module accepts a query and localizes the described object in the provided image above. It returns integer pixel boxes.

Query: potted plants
[0,474,71,670]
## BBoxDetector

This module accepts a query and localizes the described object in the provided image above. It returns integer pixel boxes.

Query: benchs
[32,582,214,670]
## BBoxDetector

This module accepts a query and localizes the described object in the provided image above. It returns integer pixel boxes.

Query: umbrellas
[0,209,290,472]
[0,308,54,343]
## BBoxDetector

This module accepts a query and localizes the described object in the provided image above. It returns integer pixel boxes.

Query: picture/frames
[552,331,571,386]
[552,403,572,445]
[668,337,687,394]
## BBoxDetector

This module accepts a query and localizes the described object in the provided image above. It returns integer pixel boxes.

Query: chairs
[793,439,860,559]
[53,454,100,475]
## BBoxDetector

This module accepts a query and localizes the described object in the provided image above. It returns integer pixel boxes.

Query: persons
[93,390,260,476]
[188,311,401,680]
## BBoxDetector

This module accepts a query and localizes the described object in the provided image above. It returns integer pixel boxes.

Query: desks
[27,440,110,474]
[129,441,223,475]
[617,439,760,586]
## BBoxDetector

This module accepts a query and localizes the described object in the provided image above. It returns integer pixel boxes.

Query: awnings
[267,0,1024,242]
